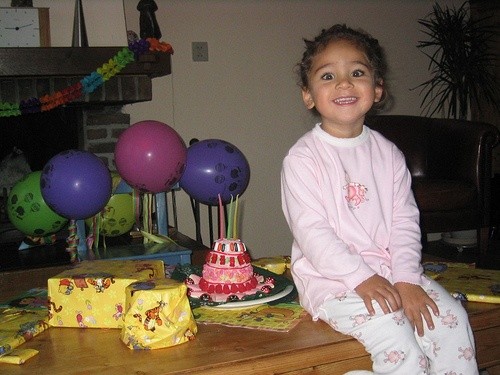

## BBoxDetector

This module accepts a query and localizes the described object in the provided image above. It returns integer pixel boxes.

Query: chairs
[364,115,500,264]
[76,174,192,265]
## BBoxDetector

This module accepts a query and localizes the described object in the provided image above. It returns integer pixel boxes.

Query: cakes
[186,237,275,302]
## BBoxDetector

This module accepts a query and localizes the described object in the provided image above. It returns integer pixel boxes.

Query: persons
[279,24,479,375]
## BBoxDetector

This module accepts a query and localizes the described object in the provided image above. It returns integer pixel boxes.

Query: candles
[218,193,239,238]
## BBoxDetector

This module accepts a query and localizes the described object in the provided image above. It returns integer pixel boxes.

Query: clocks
[0,7,51,47]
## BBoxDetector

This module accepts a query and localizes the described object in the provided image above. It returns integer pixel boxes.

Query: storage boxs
[48,259,164,328]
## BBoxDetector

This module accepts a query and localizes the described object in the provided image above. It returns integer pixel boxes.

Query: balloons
[115,121,186,193]
[83,171,140,237]
[180,138,251,206]
[7,171,68,237]
[40,150,112,219]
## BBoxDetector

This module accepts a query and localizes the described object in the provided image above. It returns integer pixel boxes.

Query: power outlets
[192,41,209,62]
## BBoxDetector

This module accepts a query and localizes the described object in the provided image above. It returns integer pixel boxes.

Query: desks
[0,267,500,375]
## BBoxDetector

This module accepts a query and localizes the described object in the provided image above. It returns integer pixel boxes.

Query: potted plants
[409,0,500,262]
